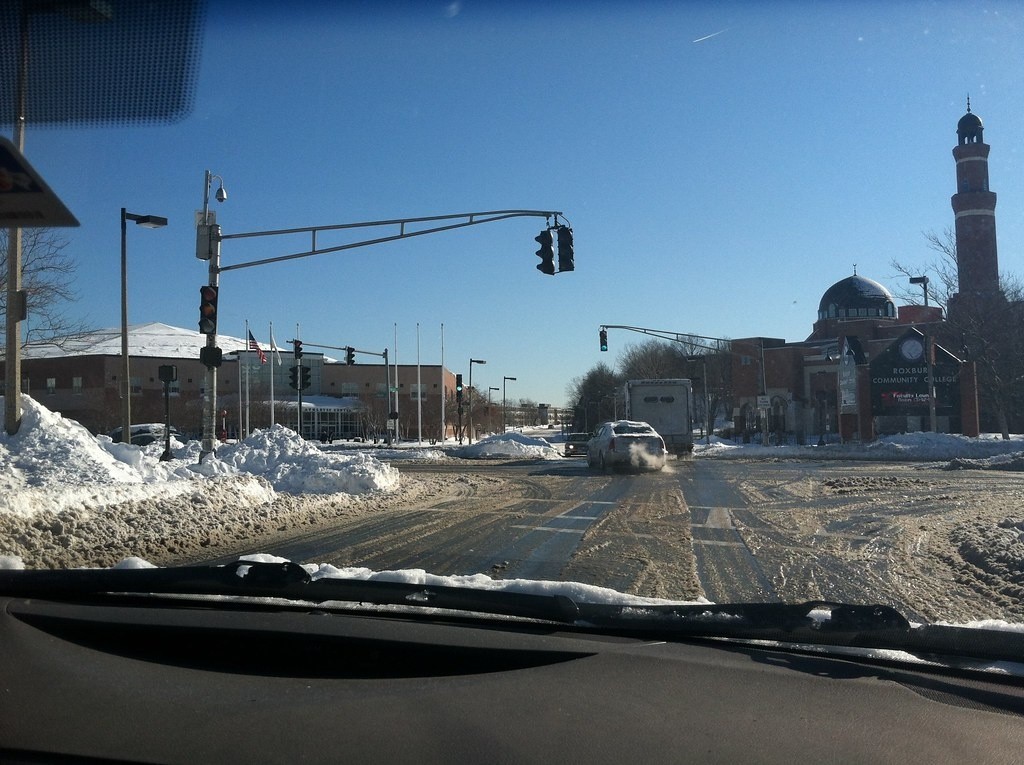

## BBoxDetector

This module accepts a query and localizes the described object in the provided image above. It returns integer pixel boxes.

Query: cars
[585,420,668,472]
[105,422,180,445]
[564,432,591,457]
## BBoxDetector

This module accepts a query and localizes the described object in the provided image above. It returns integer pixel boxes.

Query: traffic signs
[456,374,462,402]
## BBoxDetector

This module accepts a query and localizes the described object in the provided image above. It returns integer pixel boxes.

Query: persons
[321,430,333,444]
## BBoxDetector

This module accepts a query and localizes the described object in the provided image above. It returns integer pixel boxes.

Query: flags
[271,335,282,366]
[249,330,267,363]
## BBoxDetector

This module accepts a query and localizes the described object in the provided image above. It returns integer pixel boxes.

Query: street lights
[119,207,168,445]
[489,387,500,436]
[908,275,938,432]
[685,356,711,444]
[503,376,516,433]
[469,358,486,444]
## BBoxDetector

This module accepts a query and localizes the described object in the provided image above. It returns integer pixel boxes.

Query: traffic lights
[599,330,607,351]
[348,347,355,365]
[534,231,555,275]
[289,366,298,389]
[293,339,303,359]
[200,347,222,367]
[302,365,312,390]
[391,412,399,419]
[198,285,218,334]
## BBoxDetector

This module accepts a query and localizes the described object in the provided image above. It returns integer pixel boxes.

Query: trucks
[624,378,694,453]
